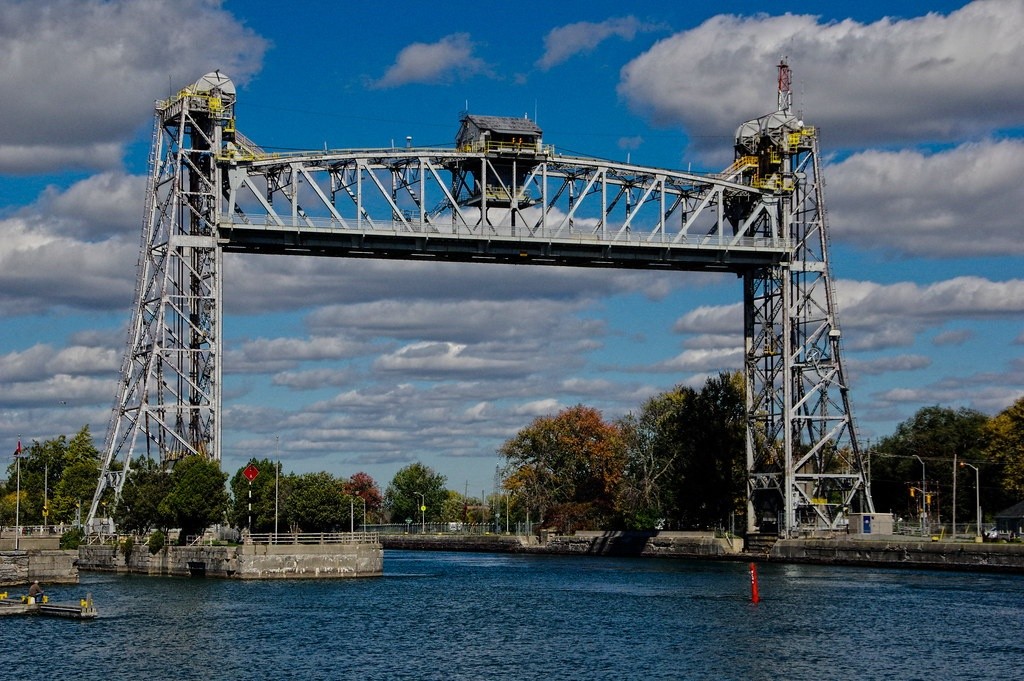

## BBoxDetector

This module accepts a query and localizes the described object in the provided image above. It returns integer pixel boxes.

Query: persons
[29,580,44,602]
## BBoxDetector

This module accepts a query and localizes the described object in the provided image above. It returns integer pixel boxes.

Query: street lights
[912,455,926,534]
[502,491,508,532]
[960,462,980,537]
[414,491,424,532]
[357,496,366,532]
[984,526,998,539]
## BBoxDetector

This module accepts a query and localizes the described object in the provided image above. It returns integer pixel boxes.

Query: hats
[34,580,39,583]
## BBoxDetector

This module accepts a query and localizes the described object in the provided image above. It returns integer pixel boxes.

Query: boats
[21,591,45,602]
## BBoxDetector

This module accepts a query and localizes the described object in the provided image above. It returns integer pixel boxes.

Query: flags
[14,440,21,460]
